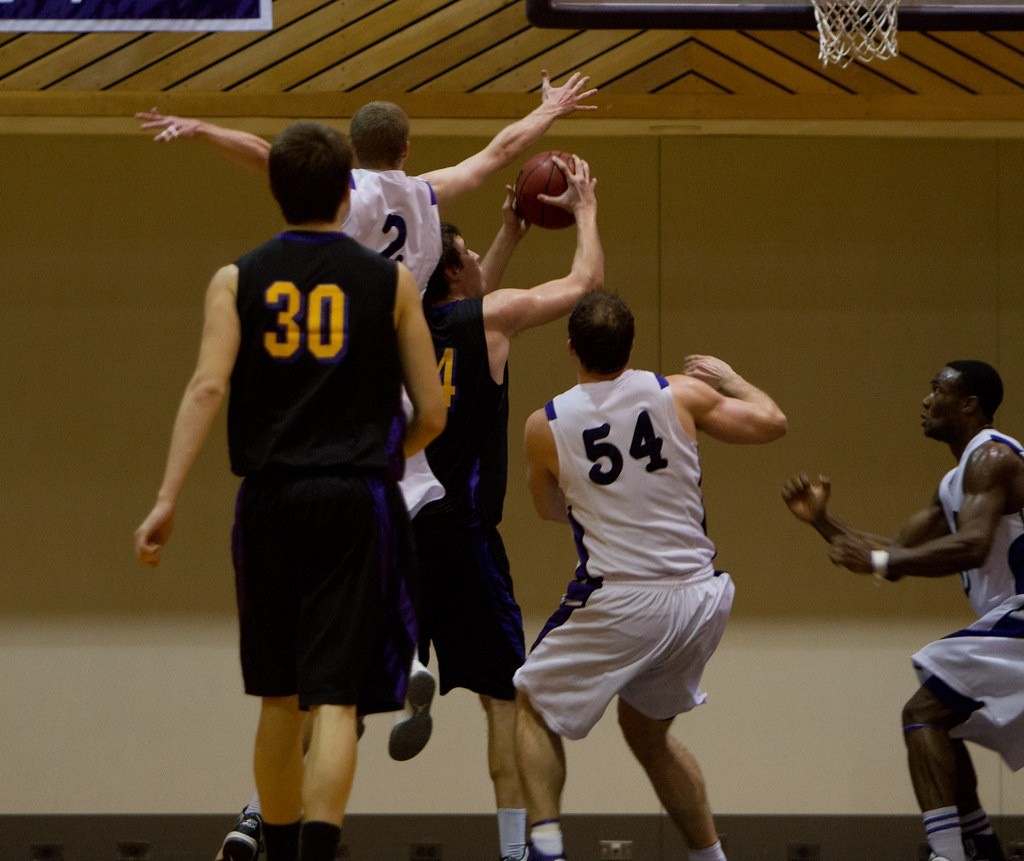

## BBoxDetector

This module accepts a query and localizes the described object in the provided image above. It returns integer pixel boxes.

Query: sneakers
[215,806,263,861]
[389,659,434,761]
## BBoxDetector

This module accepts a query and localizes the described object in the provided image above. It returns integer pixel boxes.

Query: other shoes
[956,834,1005,861]
[527,842,565,861]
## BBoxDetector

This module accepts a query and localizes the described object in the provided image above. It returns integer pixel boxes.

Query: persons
[134,124,449,861]
[134,69,599,763]
[512,288,788,861]
[782,360,1024,861]
[206,154,605,860]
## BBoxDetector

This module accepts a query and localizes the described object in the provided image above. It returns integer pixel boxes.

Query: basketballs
[515,149,592,230]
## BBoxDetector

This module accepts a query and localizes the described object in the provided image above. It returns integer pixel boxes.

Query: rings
[161,124,179,143]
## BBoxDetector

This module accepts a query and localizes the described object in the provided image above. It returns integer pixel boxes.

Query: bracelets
[871,550,890,576]
